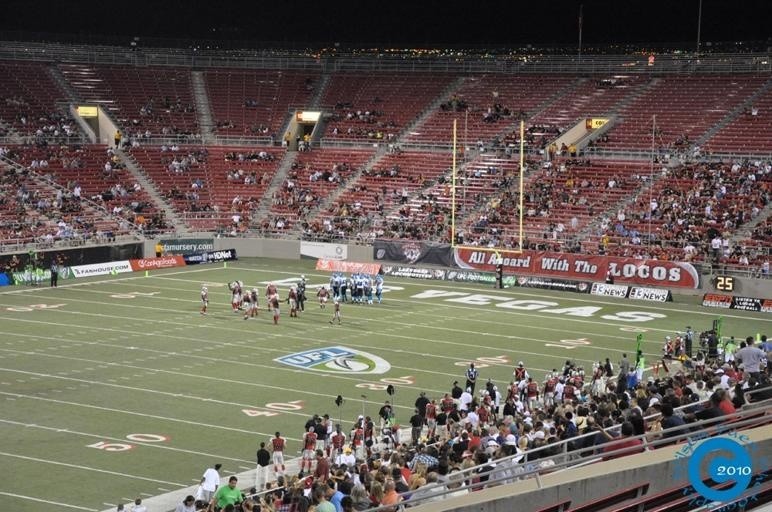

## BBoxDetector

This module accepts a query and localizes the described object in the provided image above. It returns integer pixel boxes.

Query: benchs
[1,57,769,276]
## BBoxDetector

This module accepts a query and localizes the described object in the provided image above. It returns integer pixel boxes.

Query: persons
[0,78,772,512]
